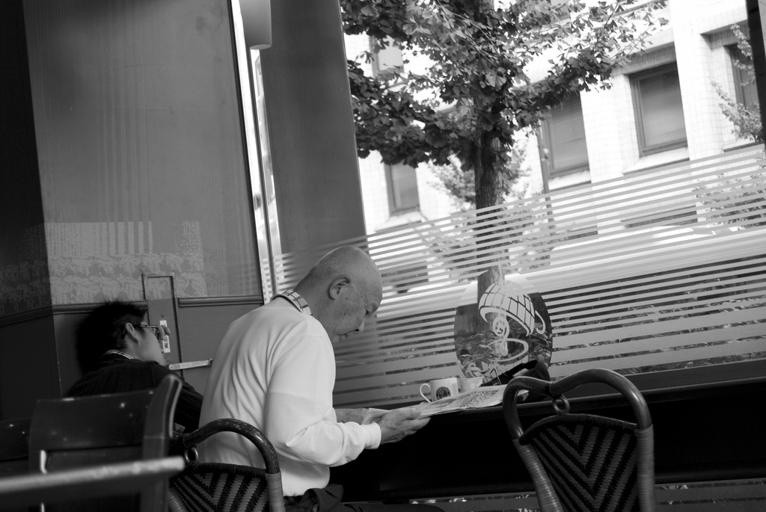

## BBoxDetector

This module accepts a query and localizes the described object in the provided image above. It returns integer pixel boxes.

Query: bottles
[157,318,174,362]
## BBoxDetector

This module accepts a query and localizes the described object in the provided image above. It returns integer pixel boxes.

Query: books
[361,384,530,423]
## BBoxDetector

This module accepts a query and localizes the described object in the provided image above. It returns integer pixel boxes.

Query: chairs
[504,369,658,511]
[1,374,286,512]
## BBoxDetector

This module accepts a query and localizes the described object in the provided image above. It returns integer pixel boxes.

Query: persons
[193,245,432,512]
[62,298,204,433]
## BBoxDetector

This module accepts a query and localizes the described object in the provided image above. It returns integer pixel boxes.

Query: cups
[460,376,484,392]
[417,377,458,404]
